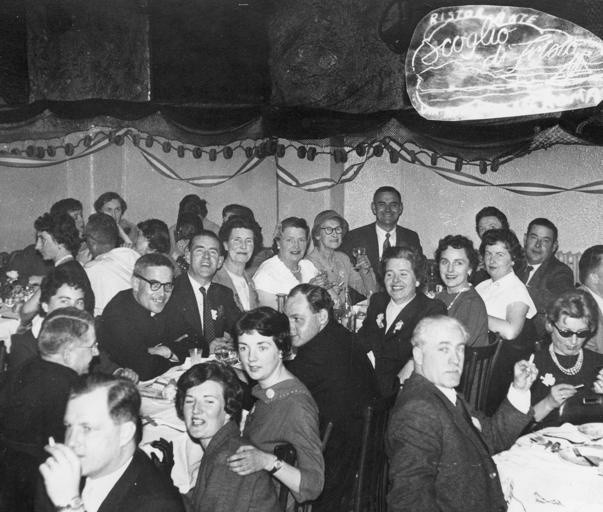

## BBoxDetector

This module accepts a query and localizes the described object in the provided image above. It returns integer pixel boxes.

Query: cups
[187,348,202,367]
[353,247,365,256]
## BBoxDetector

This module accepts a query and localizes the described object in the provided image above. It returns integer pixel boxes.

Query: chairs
[272,377,399,511]
[458,330,503,409]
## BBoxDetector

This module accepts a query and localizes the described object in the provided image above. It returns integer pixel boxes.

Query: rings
[562,395,565,399]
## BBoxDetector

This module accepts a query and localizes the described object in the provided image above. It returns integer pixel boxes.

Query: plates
[578,422,603,437]
[558,445,603,468]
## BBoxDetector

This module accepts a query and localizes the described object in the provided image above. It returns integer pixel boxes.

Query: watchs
[269,457,283,474]
[56,496,83,512]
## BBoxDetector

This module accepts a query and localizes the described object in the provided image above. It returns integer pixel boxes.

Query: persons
[175,359,278,512]
[508,295,602,430]
[2,305,98,512]
[435,233,488,406]
[225,306,324,512]
[284,284,376,512]
[0,187,602,382]
[386,315,538,512]
[39,373,179,512]
[357,247,448,401]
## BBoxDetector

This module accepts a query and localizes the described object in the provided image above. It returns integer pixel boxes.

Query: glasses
[134,272,175,292]
[551,320,589,338]
[319,225,344,235]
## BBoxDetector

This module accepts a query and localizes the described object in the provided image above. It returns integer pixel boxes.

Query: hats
[311,210,348,238]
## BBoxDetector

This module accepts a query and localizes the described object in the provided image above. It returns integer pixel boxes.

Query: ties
[521,265,533,285]
[199,286,216,343]
[455,394,488,453]
[382,234,390,254]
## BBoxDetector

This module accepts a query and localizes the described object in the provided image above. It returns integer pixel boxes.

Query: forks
[543,433,585,445]
[573,447,598,467]
